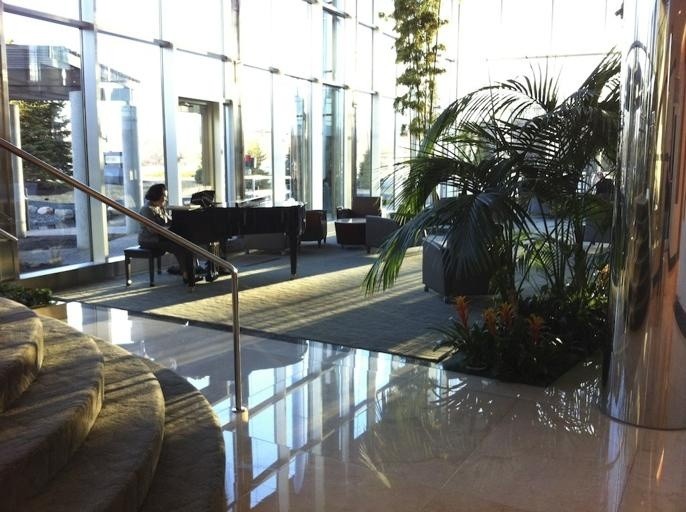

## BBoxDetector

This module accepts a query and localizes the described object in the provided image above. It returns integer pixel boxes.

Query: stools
[124,245,162,287]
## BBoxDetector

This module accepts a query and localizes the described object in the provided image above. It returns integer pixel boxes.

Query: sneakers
[183,275,203,286]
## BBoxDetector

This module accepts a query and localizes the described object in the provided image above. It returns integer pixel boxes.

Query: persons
[138,183,203,283]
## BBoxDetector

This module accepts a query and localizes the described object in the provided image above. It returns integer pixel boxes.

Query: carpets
[50,245,607,362]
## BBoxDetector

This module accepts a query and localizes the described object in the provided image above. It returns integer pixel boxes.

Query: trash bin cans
[103,152,125,184]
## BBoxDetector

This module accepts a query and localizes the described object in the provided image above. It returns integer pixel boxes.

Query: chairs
[235,196,402,258]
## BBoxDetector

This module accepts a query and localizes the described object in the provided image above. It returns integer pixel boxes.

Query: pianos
[165,200,306,282]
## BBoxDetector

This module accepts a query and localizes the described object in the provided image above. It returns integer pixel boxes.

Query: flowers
[424,293,561,381]
